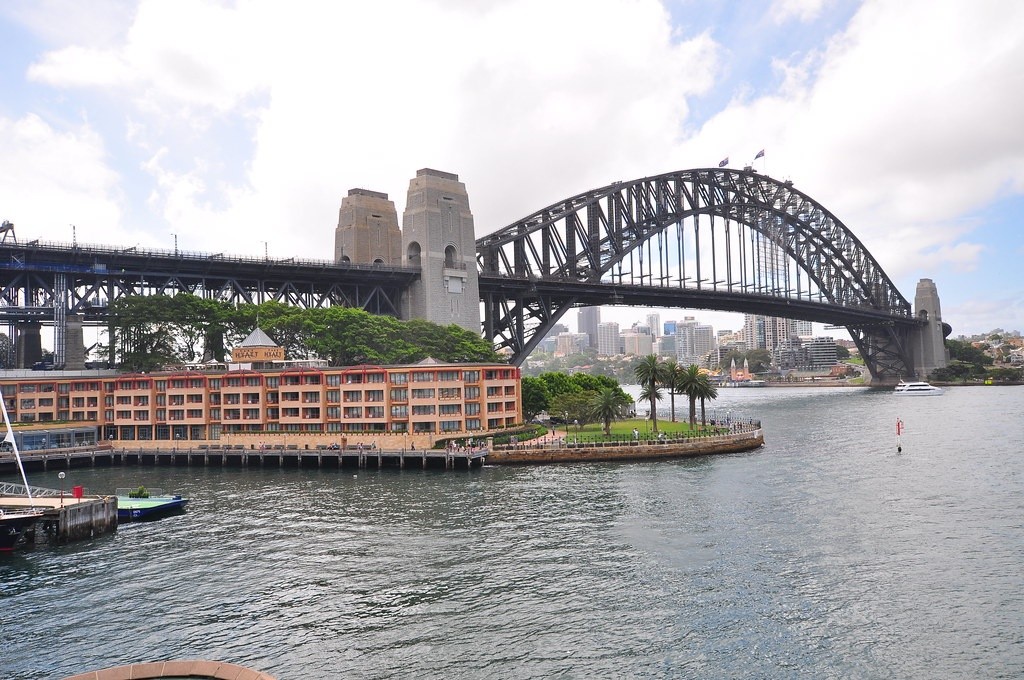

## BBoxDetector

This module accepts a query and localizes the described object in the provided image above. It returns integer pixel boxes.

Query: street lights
[403,432,407,451]
[58,472,65,508]
[645,418,648,444]
[574,419,578,447]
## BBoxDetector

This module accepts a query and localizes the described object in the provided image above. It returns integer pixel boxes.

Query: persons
[372,441,376,449]
[444,439,472,452]
[411,441,416,450]
[750,419,752,426]
[356,442,360,450]
[258,441,265,449]
[633,428,638,439]
[734,421,742,434]
[552,425,555,436]
[327,443,337,451]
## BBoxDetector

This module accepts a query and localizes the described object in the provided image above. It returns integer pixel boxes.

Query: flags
[718,157,728,167]
[755,149,764,159]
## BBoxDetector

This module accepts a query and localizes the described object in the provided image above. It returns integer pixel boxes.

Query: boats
[891,378,942,395]
